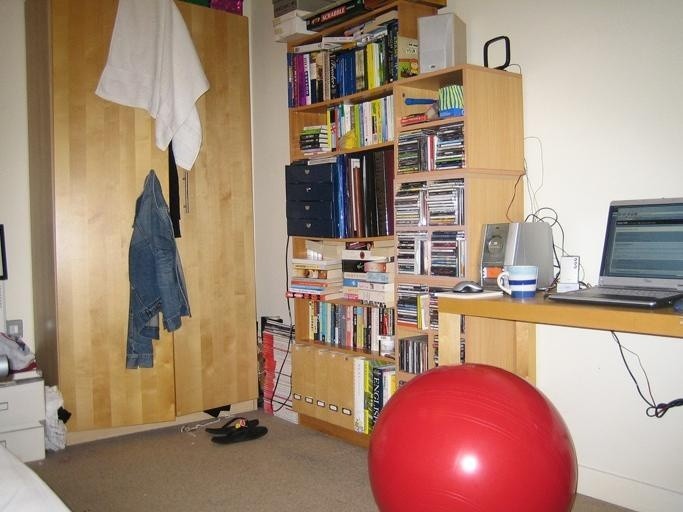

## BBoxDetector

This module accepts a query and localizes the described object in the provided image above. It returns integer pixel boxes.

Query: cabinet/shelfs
[48,0,260,431]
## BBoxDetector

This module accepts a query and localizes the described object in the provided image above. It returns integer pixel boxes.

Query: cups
[496,265,538,298]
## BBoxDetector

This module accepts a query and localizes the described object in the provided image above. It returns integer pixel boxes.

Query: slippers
[211,421,267,443]
[205,417,258,434]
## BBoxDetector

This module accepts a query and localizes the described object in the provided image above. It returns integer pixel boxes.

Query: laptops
[549,197,683,306]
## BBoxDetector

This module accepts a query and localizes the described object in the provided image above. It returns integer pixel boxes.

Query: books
[283,9,467,434]
[434,288,504,300]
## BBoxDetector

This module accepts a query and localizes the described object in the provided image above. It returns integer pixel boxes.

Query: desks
[436,281,683,370]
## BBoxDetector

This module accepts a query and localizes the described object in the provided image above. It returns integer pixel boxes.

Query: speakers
[415,13,466,75]
[479,221,554,288]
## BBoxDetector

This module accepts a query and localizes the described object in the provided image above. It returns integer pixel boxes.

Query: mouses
[451,280,484,293]
